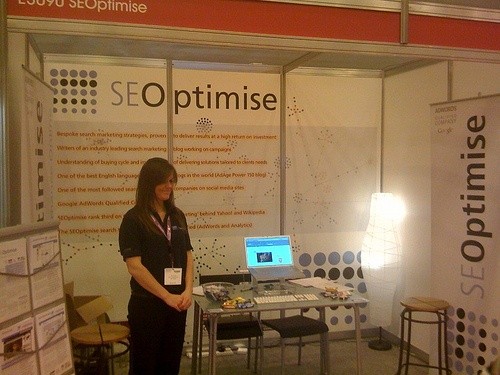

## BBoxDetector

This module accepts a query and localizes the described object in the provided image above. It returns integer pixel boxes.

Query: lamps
[358,192,405,350]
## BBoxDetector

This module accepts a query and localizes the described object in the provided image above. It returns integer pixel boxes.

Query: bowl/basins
[202,282,235,303]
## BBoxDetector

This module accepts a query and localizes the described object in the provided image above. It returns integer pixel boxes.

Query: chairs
[199,273,329,375]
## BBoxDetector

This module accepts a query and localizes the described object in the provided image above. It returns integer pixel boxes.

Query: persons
[118,158,196,375]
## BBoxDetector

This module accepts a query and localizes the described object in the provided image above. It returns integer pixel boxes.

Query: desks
[192,281,369,375]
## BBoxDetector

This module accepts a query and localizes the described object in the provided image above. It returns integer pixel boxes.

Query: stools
[70,323,130,375]
[396,296,451,375]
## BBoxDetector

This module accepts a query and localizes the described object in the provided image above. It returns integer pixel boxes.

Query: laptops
[244,235,306,282]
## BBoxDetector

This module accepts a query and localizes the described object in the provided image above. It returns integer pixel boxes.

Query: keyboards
[253,293,319,305]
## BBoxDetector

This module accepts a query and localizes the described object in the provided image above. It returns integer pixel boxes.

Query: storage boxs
[64,280,112,330]
